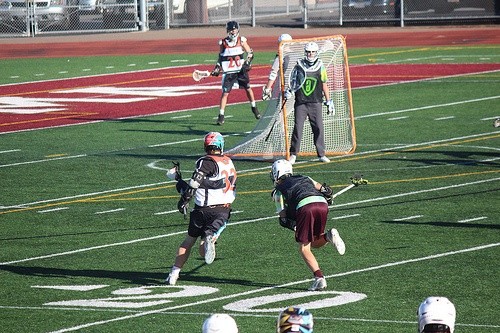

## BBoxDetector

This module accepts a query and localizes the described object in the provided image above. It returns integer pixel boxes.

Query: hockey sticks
[264,58,307,142]
[166,164,188,220]
[318,40,335,54]
[192,66,251,82]
[327,178,369,201]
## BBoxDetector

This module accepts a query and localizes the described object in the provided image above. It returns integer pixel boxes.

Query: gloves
[176,179,189,193]
[284,90,292,99]
[324,99,335,116]
[178,185,198,214]
[212,65,220,76]
[279,216,290,228]
[262,86,273,100]
[242,60,251,71]
[320,185,333,205]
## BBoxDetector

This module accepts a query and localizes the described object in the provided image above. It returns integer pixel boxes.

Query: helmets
[204,132,224,152]
[304,42,319,66]
[278,34,293,45]
[419,296,456,333]
[270,160,293,186]
[278,306,312,333]
[203,313,238,333]
[227,21,239,40]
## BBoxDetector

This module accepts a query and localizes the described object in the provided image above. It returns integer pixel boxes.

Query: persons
[417,296,456,333]
[168,131,237,287]
[211,21,262,125]
[277,307,313,333]
[269,159,345,292]
[284,42,335,165]
[262,34,300,135]
[202,314,238,333]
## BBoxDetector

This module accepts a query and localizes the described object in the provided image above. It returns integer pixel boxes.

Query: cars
[0,1,102,29]
[102,0,165,30]
[338,0,500,19]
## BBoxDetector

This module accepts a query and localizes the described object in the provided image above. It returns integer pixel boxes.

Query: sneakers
[319,155,330,162]
[289,155,296,164]
[308,276,327,290]
[217,115,224,124]
[168,269,179,285]
[204,235,215,264]
[252,106,261,119]
[327,227,345,256]
